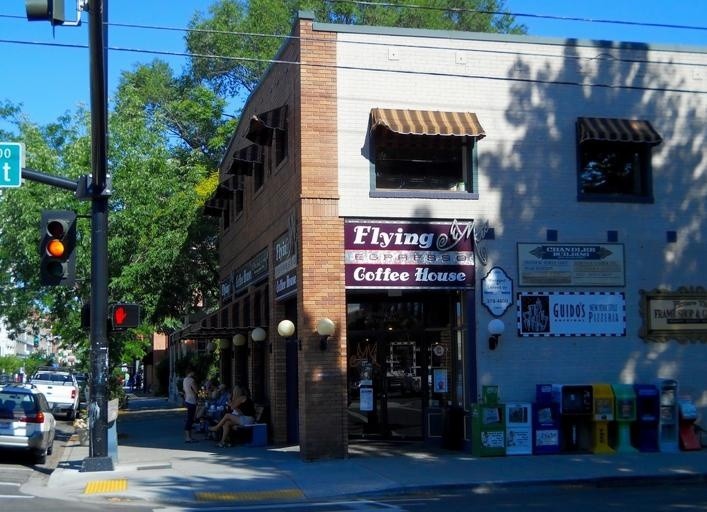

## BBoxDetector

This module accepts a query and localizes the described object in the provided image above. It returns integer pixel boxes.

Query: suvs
[0,367,87,466]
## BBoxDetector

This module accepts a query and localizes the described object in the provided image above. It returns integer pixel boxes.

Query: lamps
[488,317,504,350]
[205,317,336,359]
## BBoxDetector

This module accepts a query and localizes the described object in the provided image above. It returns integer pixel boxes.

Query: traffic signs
[0,142,22,189]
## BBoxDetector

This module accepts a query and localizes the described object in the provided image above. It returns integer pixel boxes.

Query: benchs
[200,390,268,447]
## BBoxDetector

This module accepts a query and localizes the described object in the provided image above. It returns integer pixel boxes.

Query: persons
[134,371,141,393]
[181,363,257,448]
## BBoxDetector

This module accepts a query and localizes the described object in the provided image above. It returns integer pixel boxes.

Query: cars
[348,371,433,436]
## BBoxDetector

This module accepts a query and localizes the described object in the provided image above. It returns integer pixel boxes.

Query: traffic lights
[109,302,141,331]
[24,0,62,27]
[38,206,79,290]
